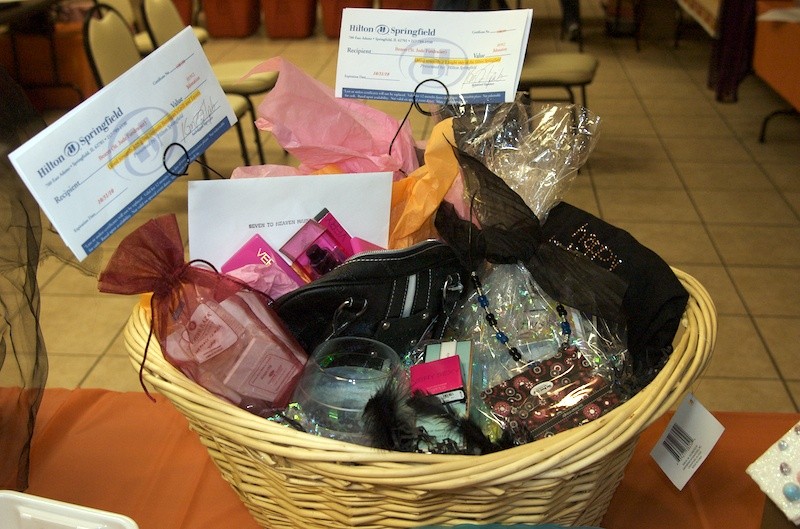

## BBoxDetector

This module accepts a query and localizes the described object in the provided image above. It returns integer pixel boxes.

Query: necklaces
[469,188,570,365]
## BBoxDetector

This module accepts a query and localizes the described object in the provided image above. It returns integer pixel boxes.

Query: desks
[0,386,800,528]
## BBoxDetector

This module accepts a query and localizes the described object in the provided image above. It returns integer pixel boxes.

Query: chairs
[480,0,599,135]
[81,0,279,189]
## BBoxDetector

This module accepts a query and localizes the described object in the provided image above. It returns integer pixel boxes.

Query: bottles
[295,335,405,448]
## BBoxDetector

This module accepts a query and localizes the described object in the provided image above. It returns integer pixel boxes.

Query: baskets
[124,265,720,529]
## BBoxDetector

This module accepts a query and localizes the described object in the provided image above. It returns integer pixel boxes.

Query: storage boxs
[410,356,466,405]
[207,289,308,401]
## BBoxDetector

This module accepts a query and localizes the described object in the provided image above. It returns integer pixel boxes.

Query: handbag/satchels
[265,240,473,368]
